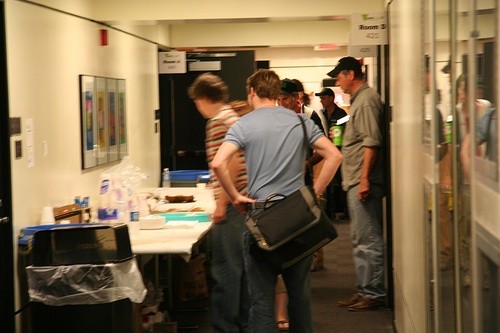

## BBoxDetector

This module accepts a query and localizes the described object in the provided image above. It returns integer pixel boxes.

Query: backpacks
[244,185,338,271]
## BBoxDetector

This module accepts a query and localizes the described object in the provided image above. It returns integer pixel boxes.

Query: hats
[327,57,361,77]
[315,88,334,96]
[279,78,298,95]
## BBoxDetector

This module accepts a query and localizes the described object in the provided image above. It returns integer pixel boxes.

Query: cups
[331,123,344,147]
[444,122,452,143]
[196,183,206,193]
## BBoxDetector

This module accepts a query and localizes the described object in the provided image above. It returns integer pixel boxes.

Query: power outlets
[16,140,22,158]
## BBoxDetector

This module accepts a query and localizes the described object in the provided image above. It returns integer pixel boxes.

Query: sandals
[276,319,290,331]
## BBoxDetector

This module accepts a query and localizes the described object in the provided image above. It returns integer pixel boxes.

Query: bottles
[130,198,141,232]
[163,168,171,192]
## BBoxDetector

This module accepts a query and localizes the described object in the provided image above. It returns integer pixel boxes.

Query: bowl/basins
[138,216,165,229]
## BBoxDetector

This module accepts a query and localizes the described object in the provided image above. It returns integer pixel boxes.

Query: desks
[93,187,216,313]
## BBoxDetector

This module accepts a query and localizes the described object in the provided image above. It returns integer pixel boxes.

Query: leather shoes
[337,295,363,307]
[347,296,384,311]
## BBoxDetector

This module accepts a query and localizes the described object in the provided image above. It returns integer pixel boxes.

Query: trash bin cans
[17,223,145,333]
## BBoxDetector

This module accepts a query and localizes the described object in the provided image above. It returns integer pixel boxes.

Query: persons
[423,56,499,311]
[277,78,327,137]
[212,69,344,333]
[272,77,327,333]
[188,74,248,332]
[289,78,326,137]
[327,57,389,311]
[312,88,348,224]
